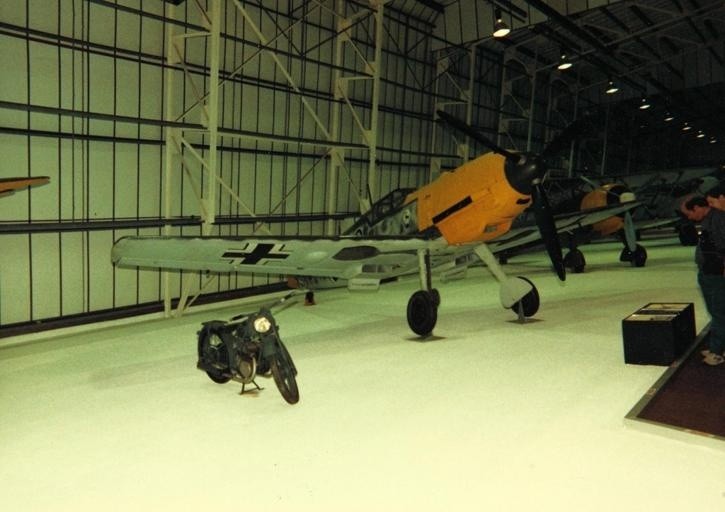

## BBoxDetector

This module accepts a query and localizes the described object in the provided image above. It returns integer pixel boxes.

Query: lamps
[493,1,618,93]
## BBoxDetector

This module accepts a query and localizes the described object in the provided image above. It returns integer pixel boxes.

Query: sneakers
[700,350,725,366]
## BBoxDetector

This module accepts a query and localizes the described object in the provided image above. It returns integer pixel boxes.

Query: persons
[681,195,725,365]
[705,184,725,208]
